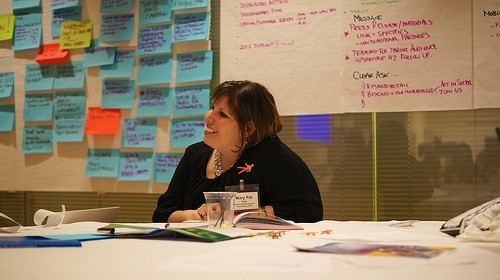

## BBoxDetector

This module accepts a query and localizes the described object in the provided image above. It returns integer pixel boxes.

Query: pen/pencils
[164,223,170,229]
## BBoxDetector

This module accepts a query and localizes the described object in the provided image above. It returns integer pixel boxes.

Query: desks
[0,220,500,280]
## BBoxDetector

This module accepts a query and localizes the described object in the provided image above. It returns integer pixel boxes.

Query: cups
[204,192,237,228]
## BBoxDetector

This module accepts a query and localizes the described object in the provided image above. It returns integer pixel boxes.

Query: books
[183,212,304,231]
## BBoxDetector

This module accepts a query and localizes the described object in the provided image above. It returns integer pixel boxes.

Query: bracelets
[258,209,266,216]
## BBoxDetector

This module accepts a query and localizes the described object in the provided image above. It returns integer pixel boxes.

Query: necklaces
[214,149,236,177]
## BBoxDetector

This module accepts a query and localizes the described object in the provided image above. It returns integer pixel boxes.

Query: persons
[152,79,324,223]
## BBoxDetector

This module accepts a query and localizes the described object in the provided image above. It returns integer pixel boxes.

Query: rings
[200,214,205,219]
